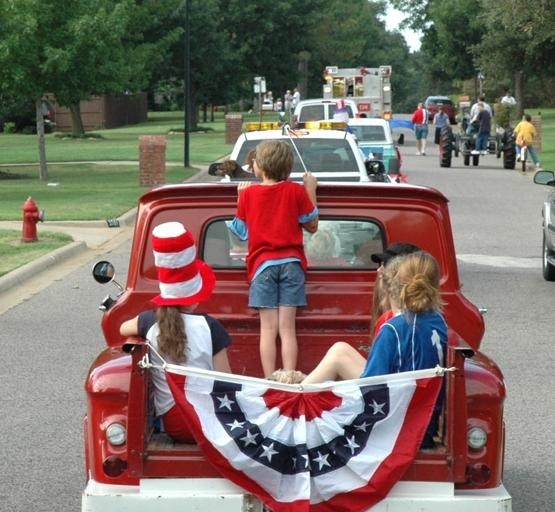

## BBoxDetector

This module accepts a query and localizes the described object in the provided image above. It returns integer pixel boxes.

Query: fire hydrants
[19,193,46,244]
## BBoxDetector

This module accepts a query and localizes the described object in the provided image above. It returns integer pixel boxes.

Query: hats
[370,244,423,262]
[148,222,216,307]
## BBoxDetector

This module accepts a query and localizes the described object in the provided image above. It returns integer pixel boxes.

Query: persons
[292,88,300,108]
[228,138,319,380]
[263,92,272,104]
[284,90,292,109]
[411,87,540,171]
[276,97,283,111]
[119,220,233,444]
[299,250,448,450]
[370,240,421,339]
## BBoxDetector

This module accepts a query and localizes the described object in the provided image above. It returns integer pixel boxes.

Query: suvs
[22,100,57,133]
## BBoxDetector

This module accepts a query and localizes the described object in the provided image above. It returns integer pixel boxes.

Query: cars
[533,168,555,280]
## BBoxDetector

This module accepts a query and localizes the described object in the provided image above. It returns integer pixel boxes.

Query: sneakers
[415,152,420,155]
[516,154,520,161]
[471,149,480,154]
[482,150,487,155]
[422,151,425,155]
[535,163,540,167]
